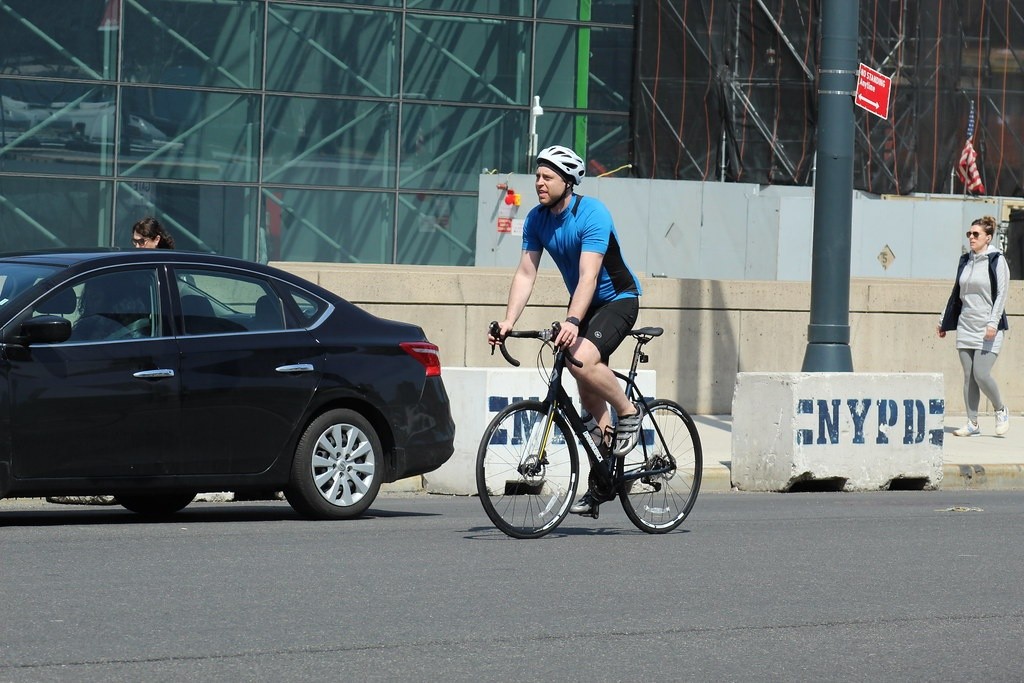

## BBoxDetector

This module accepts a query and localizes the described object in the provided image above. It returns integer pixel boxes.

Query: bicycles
[475,321,704,539]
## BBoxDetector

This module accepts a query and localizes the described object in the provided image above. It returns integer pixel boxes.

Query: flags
[958,104,984,195]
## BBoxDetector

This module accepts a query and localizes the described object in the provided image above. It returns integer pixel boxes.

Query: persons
[937,216,1010,437]
[131,218,175,249]
[75,273,125,340]
[488,145,641,514]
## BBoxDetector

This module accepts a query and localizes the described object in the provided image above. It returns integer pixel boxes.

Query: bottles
[581,408,604,447]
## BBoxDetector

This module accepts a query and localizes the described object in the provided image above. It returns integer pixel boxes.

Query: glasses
[967,232,988,238]
[131,234,157,246]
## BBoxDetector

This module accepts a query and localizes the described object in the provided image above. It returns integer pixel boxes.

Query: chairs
[179,295,221,334]
[247,293,294,330]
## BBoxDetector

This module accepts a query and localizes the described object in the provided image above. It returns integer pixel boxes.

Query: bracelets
[566,317,579,326]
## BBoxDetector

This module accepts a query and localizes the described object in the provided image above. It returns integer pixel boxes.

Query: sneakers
[953,422,980,436]
[570,492,607,513]
[612,401,643,456]
[995,405,1009,434]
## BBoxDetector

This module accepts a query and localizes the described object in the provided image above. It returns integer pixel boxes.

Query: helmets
[536,146,585,186]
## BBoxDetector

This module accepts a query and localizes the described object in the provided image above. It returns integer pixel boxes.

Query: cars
[0,250,455,522]
